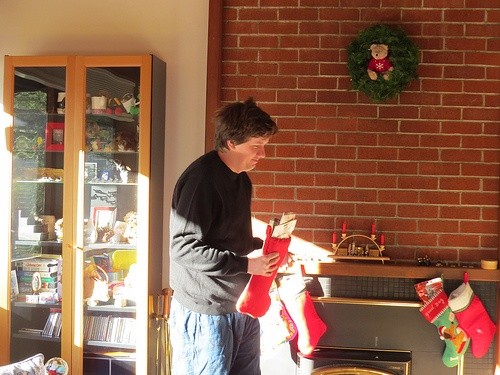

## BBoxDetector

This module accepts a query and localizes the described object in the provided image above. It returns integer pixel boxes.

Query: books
[11,212,136,349]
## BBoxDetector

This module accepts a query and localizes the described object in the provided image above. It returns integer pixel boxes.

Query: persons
[169,95,295,375]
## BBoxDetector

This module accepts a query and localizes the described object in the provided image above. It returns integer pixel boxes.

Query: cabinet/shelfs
[0,53,167,375]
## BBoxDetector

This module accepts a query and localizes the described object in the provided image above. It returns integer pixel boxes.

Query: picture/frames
[45,122,64,152]
[93,206,118,227]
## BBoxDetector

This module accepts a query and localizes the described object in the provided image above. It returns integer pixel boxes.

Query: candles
[381,233,385,245]
[372,223,375,235]
[333,231,337,244]
[342,221,347,233]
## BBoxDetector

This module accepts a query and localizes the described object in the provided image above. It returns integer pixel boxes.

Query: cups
[92,96,107,114]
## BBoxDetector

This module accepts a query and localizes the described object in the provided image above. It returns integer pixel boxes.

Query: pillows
[0,353,46,375]
